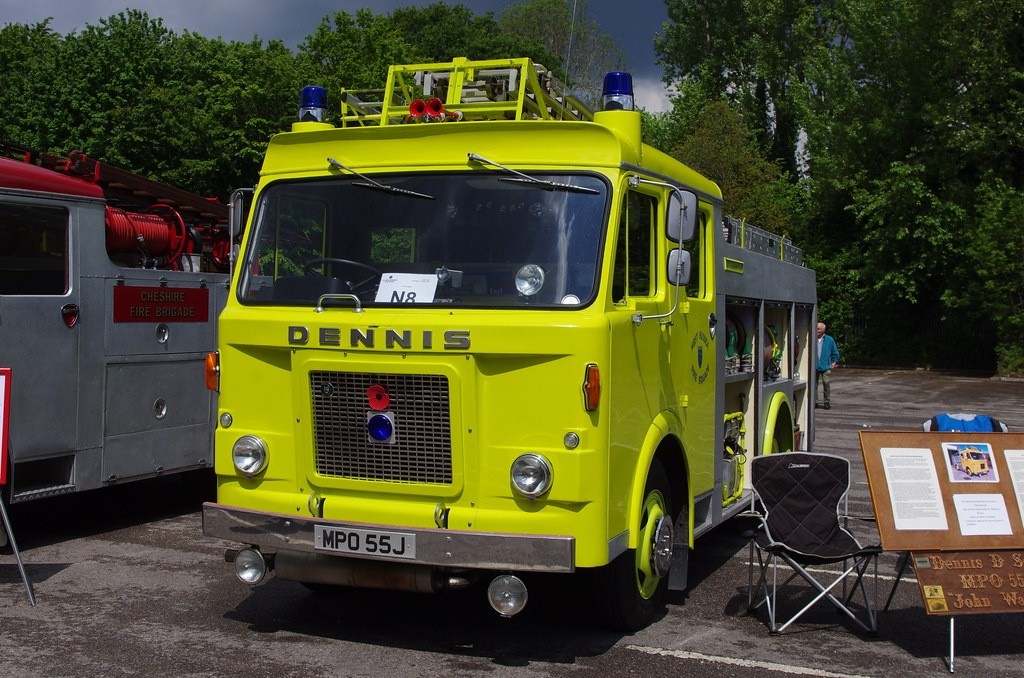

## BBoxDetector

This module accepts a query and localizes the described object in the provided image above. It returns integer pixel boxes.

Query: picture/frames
[858,430,1024,549]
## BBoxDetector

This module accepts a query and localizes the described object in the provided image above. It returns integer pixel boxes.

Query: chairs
[745,452,884,638]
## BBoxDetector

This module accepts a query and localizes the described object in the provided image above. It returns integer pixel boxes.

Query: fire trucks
[201,58,823,612]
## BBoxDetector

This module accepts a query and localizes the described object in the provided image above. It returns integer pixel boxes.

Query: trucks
[949,450,989,477]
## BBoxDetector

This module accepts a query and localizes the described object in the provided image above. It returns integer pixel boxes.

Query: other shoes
[824,402,830,409]
[815,403,819,408]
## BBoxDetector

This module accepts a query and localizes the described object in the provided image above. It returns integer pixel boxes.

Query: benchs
[348,260,595,297]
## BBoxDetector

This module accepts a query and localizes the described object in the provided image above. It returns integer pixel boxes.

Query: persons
[815,322,839,409]
